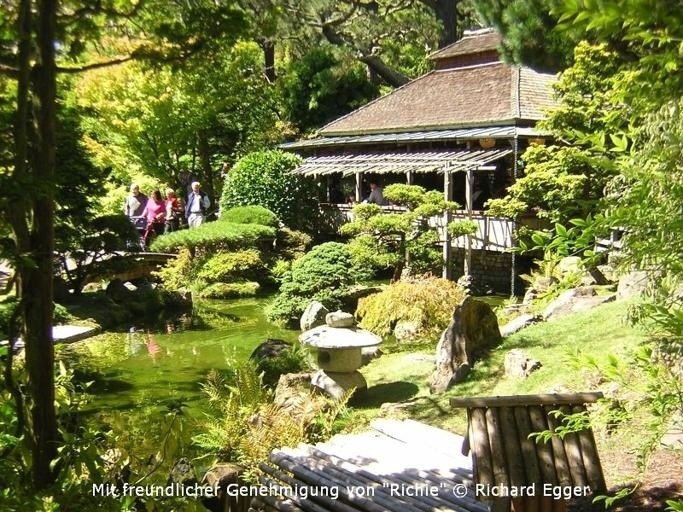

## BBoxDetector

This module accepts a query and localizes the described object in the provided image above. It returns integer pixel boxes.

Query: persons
[184,182,211,228]
[346,191,356,208]
[368,183,383,205]
[163,187,179,232]
[124,185,147,216]
[140,190,166,235]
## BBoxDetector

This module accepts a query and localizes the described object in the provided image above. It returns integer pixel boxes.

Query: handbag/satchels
[171,208,185,217]
